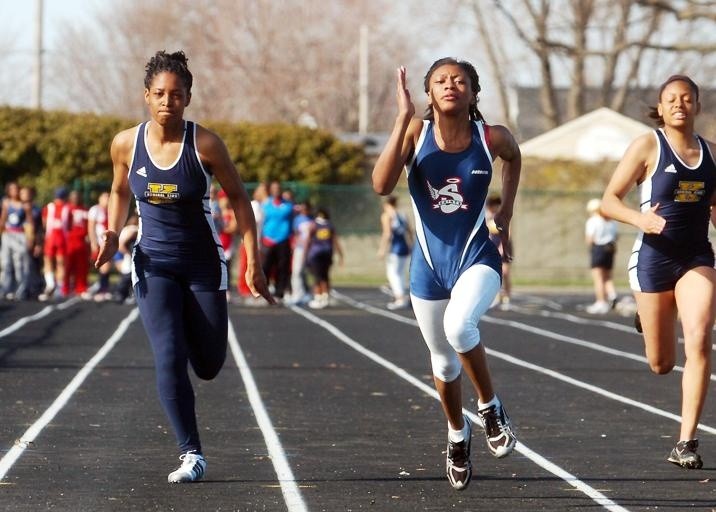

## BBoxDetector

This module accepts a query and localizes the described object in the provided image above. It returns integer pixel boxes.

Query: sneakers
[167,452,208,485]
[445,413,473,491]
[666,438,704,470]
[476,392,517,461]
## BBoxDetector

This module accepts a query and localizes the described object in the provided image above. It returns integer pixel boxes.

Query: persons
[210,180,343,308]
[487,194,511,310]
[96,49,277,485]
[0,182,139,307]
[372,58,522,490]
[600,76,716,470]
[584,198,618,314]
[378,195,413,308]
